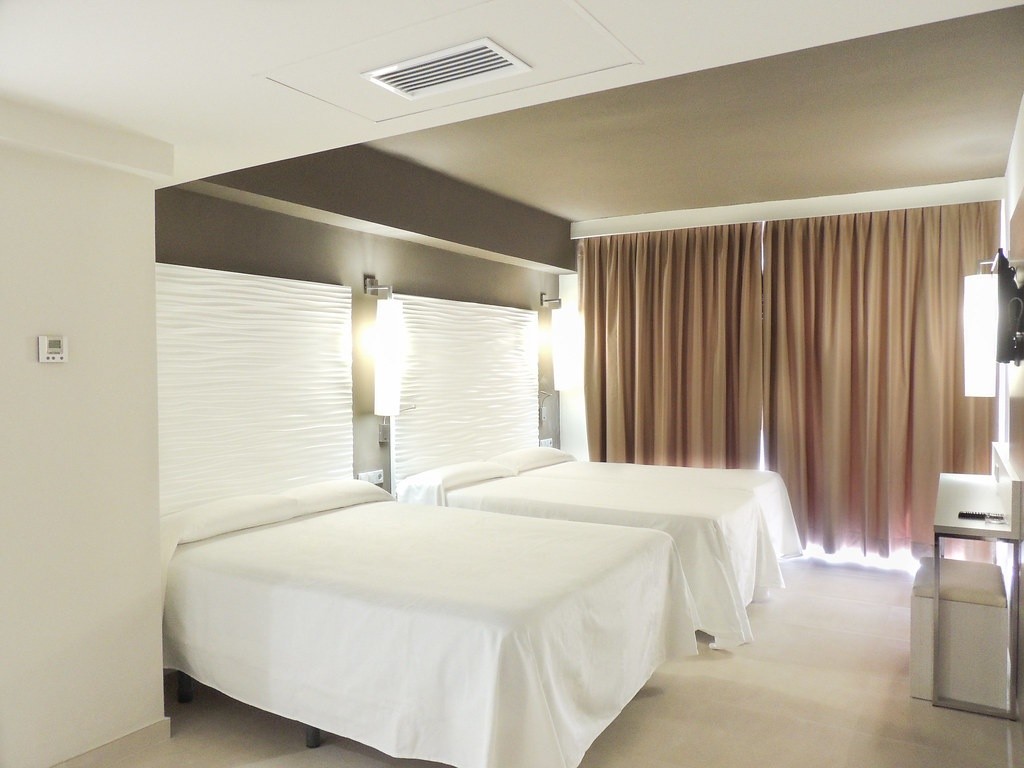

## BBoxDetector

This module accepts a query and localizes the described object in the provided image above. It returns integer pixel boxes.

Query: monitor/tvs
[995,248,1024,364]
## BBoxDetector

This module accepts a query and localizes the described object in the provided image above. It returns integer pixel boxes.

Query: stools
[909,556,1008,711]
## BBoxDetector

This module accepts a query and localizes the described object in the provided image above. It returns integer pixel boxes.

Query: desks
[933,441,1024,721]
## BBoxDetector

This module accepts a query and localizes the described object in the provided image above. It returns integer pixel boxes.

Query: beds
[160,479,699,768]
[396,446,806,651]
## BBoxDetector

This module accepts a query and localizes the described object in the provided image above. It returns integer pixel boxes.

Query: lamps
[541,292,583,391]
[366,278,404,415]
[963,259,998,398]
